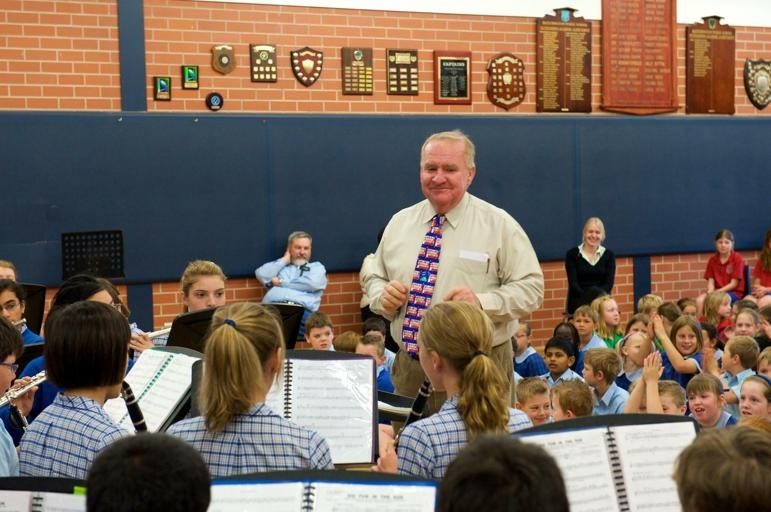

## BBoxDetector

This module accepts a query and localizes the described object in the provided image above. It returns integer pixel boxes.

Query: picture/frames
[152,73,172,103]
[432,49,477,108]
[385,46,421,98]
[180,64,200,91]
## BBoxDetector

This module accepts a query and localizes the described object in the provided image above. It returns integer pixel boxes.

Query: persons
[358,129,545,438]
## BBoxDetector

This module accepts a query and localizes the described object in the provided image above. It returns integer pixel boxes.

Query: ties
[402,215,446,361]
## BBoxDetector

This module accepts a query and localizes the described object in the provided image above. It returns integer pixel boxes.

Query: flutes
[0,369,49,408]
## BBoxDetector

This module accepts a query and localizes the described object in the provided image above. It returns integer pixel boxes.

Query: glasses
[0,362,19,373]
